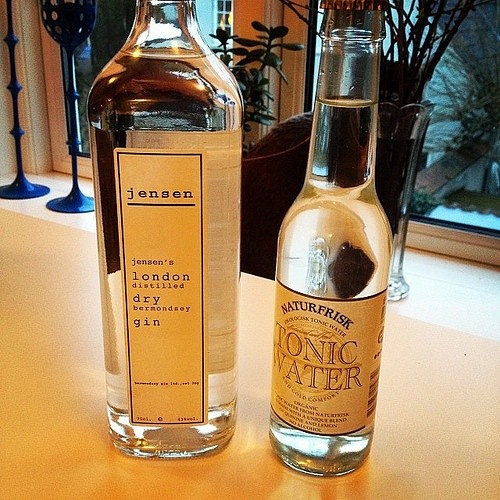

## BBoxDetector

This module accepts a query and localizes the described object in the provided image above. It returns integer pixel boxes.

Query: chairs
[242,62,432,288]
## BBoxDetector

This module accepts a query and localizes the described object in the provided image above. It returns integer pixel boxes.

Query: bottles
[269,0,396,476]
[86,0,245,460]
